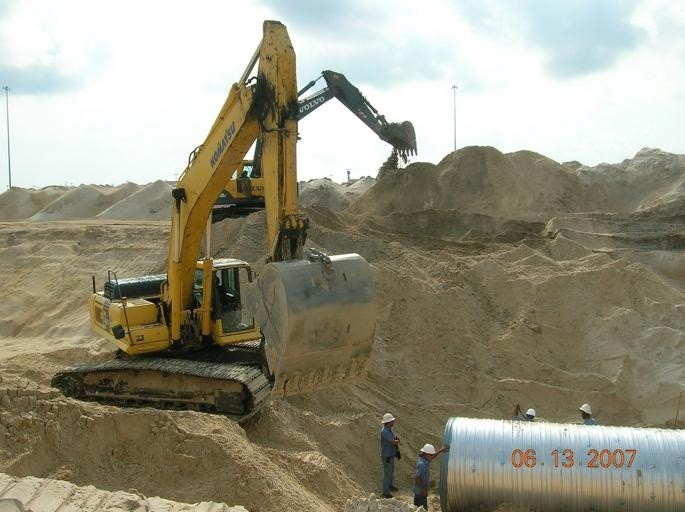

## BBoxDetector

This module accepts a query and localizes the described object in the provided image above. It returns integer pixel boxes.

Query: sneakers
[390,486,399,492]
[384,495,393,498]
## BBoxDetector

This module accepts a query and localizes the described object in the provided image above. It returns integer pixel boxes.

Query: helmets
[526,409,536,417]
[420,444,435,455]
[579,404,592,415]
[381,413,396,424]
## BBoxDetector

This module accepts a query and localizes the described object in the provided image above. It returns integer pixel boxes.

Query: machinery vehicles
[212,70,418,223]
[52,19,377,424]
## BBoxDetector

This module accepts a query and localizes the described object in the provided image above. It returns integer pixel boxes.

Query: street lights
[451,85,458,151]
[3,85,11,188]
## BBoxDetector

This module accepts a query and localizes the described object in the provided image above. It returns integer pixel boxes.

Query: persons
[216,276,237,306]
[577,403,599,425]
[514,408,536,422]
[379,413,401,499]
[412,442,449,511]
